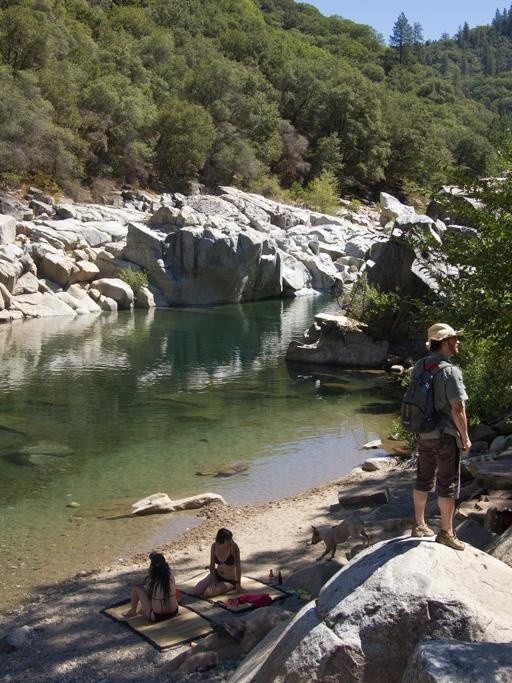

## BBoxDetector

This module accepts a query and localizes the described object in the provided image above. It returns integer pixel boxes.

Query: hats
[426,323,463,350]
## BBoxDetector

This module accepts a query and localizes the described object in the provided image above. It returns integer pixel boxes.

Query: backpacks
[401,362,446,434]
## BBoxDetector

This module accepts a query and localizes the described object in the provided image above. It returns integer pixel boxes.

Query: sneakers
[412,523,434,537]
[437,529,465,550]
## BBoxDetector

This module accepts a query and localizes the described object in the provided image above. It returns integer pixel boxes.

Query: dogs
[310,504,383,562]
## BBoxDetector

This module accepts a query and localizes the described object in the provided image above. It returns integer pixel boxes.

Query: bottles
[268,569,275,583]
[277,571,282,584]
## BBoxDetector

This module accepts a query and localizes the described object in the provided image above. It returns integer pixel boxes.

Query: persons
[196,529,248,597]
[122,553,178,623]
[411,323,472,551]
